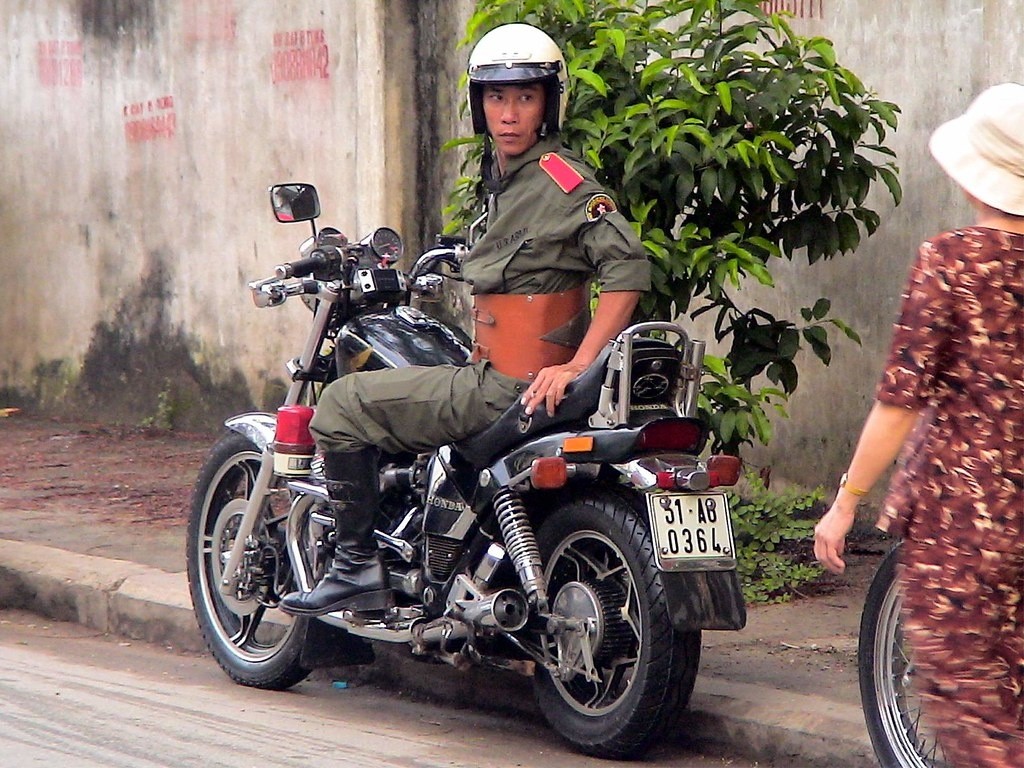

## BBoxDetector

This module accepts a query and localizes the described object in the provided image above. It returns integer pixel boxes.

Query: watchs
[840,474,869,497]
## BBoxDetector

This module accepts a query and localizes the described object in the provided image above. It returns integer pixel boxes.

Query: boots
[278,445,396,615]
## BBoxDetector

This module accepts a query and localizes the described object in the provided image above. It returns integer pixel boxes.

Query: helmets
[466,23,568,134]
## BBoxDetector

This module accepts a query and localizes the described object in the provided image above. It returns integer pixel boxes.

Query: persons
[276,24,652,617]
[814,82,1024,768]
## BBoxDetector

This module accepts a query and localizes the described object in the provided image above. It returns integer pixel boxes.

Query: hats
[928,82,1024,216]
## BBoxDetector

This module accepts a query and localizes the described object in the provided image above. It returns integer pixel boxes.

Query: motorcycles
[184,182,749,762]
